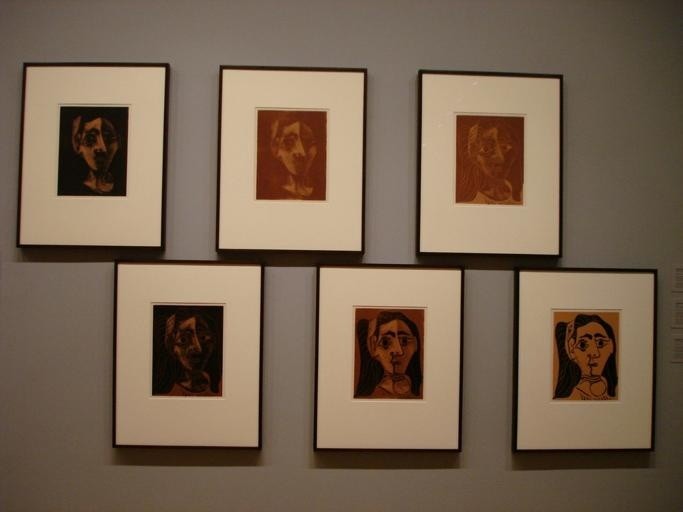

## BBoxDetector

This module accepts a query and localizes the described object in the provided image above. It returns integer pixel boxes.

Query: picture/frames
[16,61,658,454]
